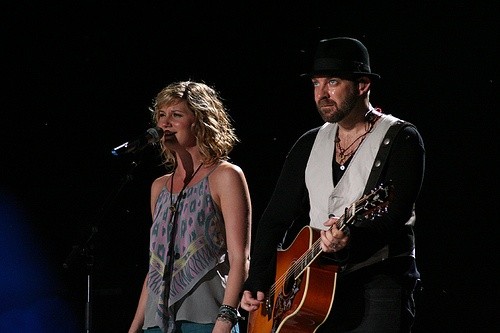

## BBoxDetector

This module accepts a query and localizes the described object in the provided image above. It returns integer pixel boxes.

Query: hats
[297,37,382,81]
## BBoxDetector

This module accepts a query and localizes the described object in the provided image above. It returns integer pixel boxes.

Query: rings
[331,243,336,249]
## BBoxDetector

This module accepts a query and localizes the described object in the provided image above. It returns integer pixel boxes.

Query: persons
[240,38,426,333]
[127,81,250,333]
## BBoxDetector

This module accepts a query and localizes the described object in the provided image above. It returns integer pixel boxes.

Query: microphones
[111,127,164,157]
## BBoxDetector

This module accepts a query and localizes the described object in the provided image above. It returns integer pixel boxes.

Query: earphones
[358,82,365,90]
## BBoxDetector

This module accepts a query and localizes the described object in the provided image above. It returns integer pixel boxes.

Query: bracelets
[217,304,238,326]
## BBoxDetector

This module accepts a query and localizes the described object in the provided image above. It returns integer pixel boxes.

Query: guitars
[247,186,389,333]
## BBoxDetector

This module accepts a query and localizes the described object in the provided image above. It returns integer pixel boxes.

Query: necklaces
[332,124,373,160]
[170,159,204,222]
